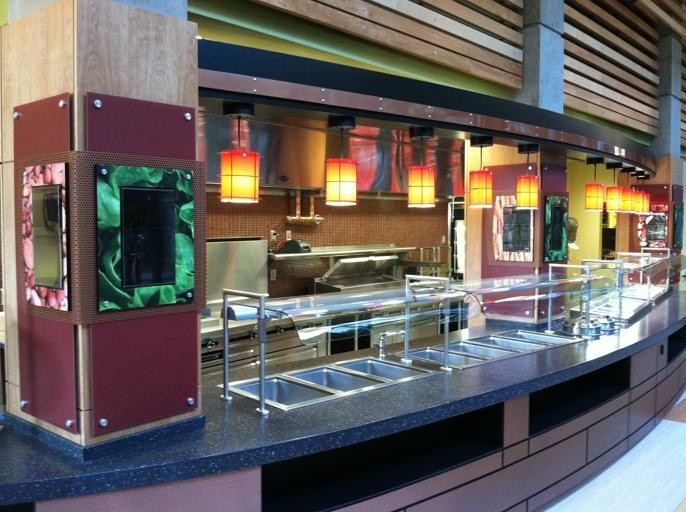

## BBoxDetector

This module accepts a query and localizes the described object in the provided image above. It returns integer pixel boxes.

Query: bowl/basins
[562,318,615,337]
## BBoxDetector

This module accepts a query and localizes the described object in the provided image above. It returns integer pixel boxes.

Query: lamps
[587,158,652,216]
[218,101,262,206]
[324,113,360,207]
[468,135,494,210]
[405,127,437,210]
[516,144,539,208]
